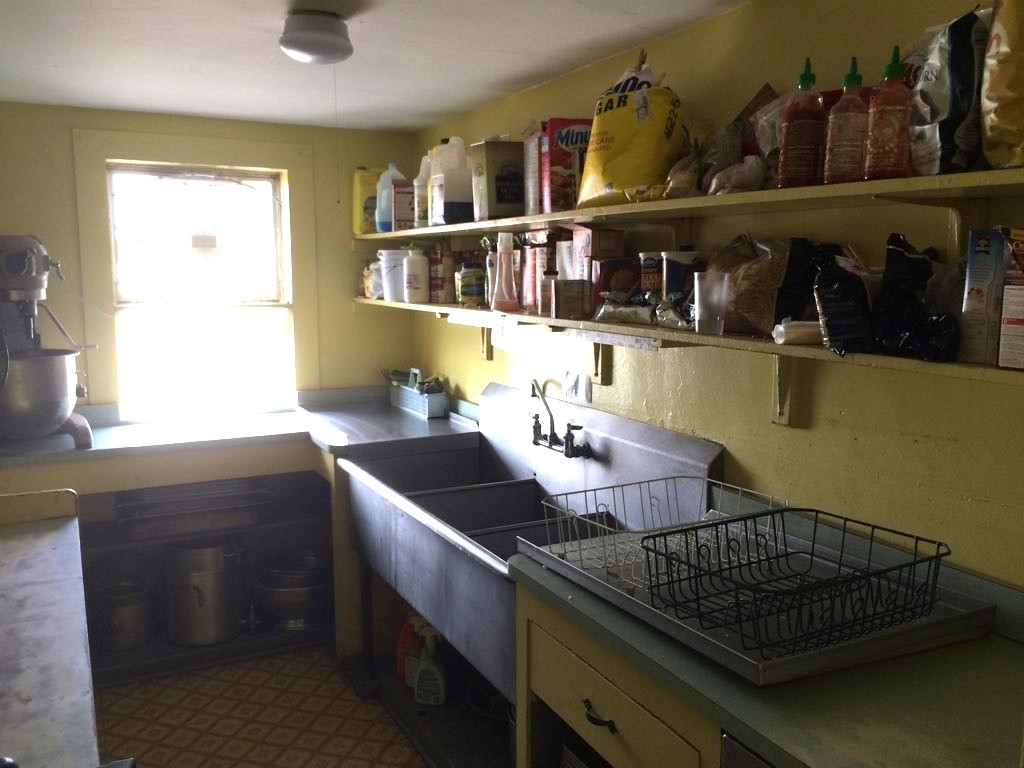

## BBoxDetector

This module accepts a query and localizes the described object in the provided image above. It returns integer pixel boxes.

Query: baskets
[541,475,789,594]
[641,504,951,659]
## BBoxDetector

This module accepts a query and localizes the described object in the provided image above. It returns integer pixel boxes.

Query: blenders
[0,235,99,448]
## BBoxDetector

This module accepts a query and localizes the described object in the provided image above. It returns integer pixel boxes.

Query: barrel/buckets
[429,248,455,303]
[160,542,245,649]
[377,248,409,301]
[89,583,155,652]
[255,550,331,629]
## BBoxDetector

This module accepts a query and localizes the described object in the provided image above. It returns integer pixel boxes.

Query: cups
[639,249,732,335]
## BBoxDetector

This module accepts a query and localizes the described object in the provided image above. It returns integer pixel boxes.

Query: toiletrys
[491,231,520,311]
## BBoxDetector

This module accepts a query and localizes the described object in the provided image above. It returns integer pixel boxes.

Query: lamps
[278,11,352,65]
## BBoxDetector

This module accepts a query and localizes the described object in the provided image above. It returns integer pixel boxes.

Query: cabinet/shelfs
[353,169,1024,389]
[81,508,333,691]
[515,586,724,768]
[0,489,102,768]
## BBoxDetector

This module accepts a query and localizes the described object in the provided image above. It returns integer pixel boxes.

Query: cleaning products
[413,627,446,706]
[395,607,431,689]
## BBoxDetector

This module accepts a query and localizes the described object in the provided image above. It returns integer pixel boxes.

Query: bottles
[412,136,472,228]
[825,56,869,184]
[400,249,431,303]
[455,232,572,315]
[863,46,914,179]
[349,162,407,234]
[777,56,825,188]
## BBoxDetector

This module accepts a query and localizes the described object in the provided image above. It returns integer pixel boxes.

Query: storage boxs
[956,230,1003,368]
[993,226,1024,372]
[555,221,642,317]
[470,119,594,221]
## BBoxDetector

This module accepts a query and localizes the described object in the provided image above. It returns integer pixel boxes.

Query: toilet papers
[771,317,823,345]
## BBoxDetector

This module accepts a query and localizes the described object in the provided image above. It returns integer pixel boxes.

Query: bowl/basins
[1,349,81,441]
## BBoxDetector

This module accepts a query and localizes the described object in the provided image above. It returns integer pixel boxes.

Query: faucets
[530,378,559,446]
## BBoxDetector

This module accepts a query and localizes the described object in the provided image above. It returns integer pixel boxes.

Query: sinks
[405,483,607,532]
[466,514,629,562]
[336,449,535,493]
